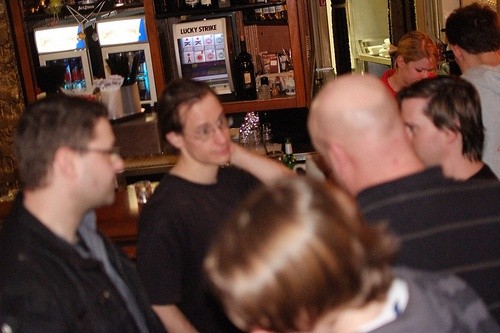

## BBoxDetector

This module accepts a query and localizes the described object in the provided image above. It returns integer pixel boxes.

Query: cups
[135,180,152,210]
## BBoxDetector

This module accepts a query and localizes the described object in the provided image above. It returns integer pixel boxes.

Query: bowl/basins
[94,82,142,122]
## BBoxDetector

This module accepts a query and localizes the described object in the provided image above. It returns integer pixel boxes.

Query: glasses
[67,142,121,163]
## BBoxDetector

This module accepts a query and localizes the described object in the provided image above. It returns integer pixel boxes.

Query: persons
[204,172,500,333]
[136,79,300,333]
[378,31,441,98]
[306,72,499,327]
[0,94,167,333]
[445,3,500,179]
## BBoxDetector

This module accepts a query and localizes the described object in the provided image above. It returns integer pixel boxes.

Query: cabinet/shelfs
[143,0,306,115]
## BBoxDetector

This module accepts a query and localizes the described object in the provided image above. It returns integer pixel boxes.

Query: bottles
[281,138,296,170]
[256,75,296,99]
[235,36,255,100]
[182,0,286,21]
